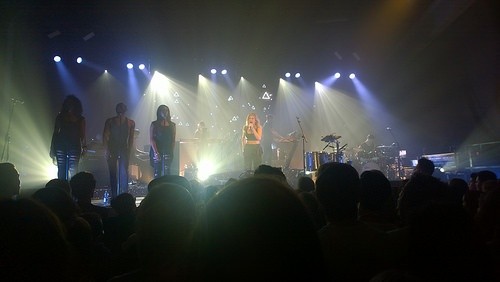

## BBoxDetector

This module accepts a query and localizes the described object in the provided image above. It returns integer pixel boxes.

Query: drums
[304,151,318,171]
[318,151,344,165]
[362,162,380,172]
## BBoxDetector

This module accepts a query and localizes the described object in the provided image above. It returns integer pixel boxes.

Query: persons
[193,121,208,161]
[149,105,176,178]
[102,103,135,200]
[49,95,88,183]
[262,114,286,169]
[134,128,149,156]
[242,112,262,172]
[0,159,498,282]
[359,134,374,151]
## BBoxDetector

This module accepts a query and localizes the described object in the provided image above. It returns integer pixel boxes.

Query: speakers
[204,184,223,193]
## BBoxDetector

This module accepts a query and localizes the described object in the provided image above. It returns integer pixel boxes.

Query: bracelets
[82,146,86,148]
[154,152,159,155]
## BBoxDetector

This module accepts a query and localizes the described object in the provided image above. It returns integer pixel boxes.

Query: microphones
[10,98,24,105]
[296,116,299,119]
[120,112,122,116]
[249,122,252,130]
[386,128,391,130]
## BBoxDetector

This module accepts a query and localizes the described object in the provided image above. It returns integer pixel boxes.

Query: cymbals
[377,146,389,148]
[353,147,364,151]
